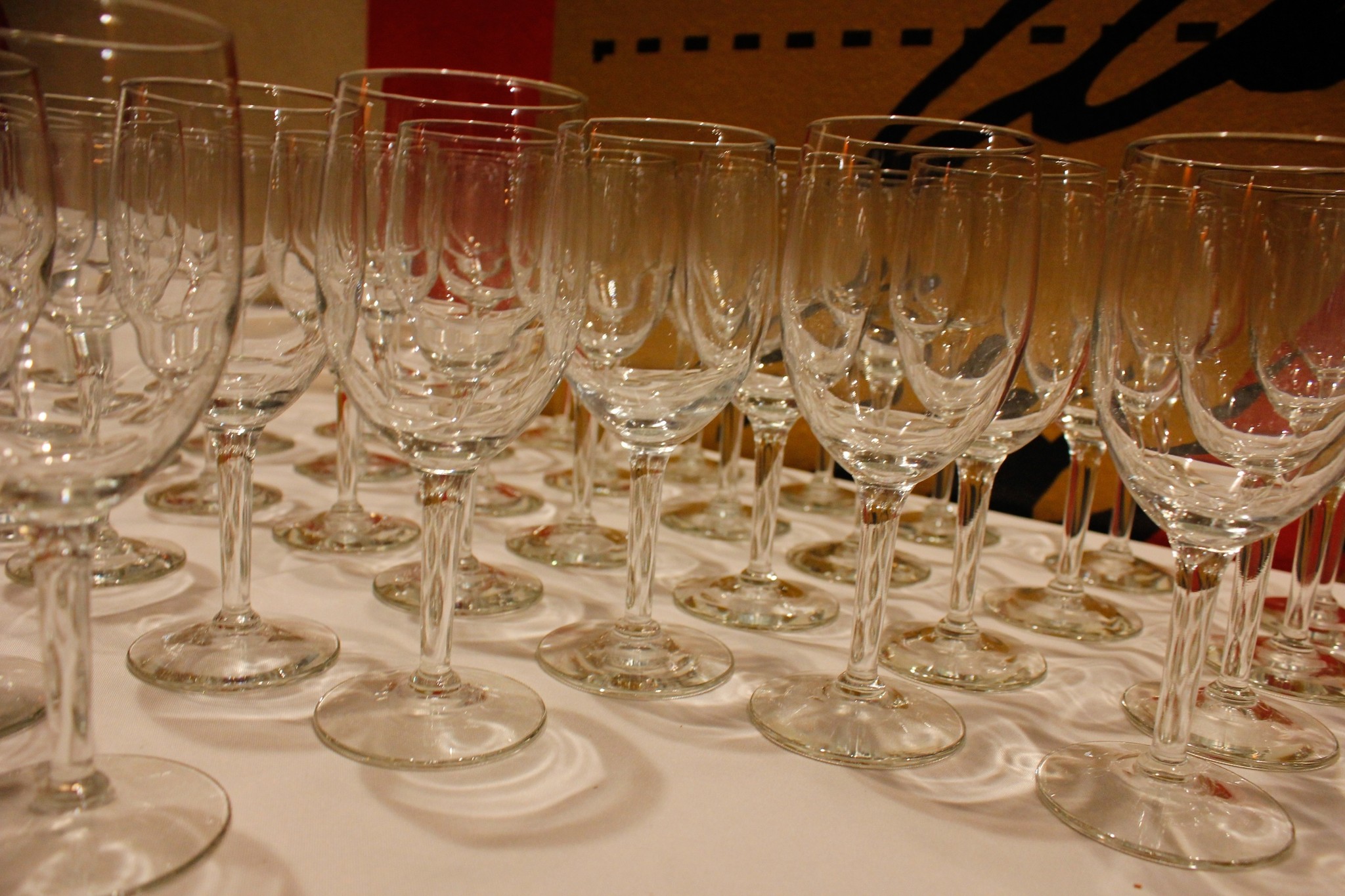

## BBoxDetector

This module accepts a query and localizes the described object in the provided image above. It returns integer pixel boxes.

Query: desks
[5,203,1345,896]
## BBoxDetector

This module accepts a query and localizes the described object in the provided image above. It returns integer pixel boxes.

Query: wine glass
[1,1,1345,893]
